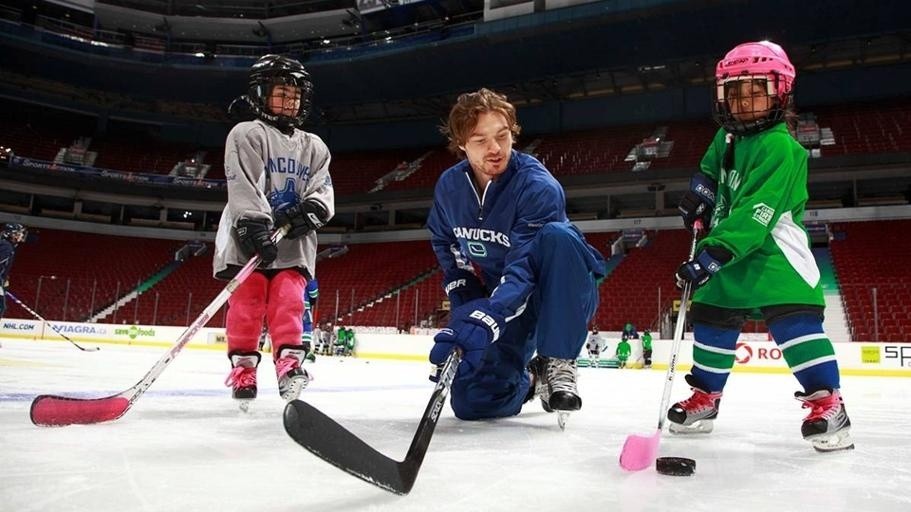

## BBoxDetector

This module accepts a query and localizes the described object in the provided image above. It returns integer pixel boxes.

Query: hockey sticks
[282,346,462,496]
[618,221,702,470]
[30,221,294,423]
[6,292,99,352]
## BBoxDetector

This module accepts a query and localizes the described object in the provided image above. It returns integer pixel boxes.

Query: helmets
[0,222,31,248]
[710,38,797,136]
[245,53,313,129]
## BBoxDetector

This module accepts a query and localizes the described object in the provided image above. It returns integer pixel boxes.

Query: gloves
[429,298,508,382]
[440,274,484,320]
[232,218,278,270]
[674,243,733,292]
[275,198,329,240]
[677,170,719,236]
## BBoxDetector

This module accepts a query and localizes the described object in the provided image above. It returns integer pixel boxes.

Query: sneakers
[666,373,722,425]
[794,383,852,441]
[532,354,556,414]
[545,356,582,412]
[272,344,309,396]
[227,350,262,399]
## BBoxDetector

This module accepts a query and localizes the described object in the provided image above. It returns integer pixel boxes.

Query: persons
[429,88,605,421]
[586,322,652,368]
[668,39,849,439]
[0,224,28,348]
[313,323,354,355]
[212,54,334,400]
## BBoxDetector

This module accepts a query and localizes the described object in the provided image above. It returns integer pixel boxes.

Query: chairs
[511,101,911,344]
[0,139,463,329]
